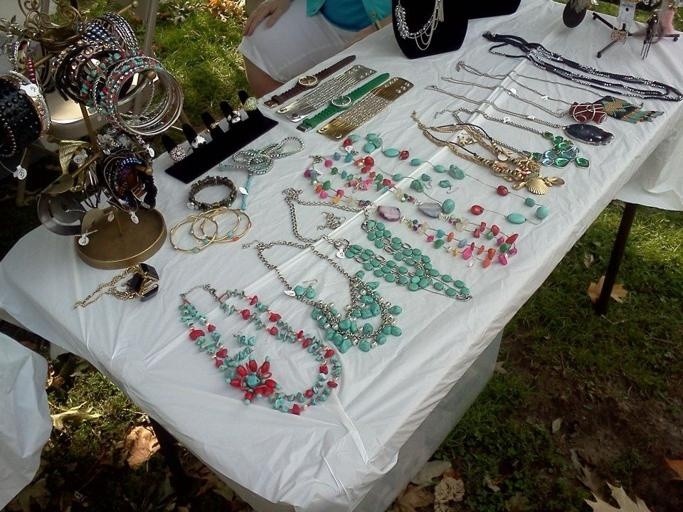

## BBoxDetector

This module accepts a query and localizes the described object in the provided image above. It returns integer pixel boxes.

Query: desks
[0,0,683,512]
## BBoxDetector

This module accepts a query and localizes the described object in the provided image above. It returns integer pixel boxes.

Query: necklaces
[75,264,159,312]
[179,1,683,416]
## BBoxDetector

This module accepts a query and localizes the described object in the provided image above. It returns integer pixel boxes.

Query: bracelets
[2,9,251,253]
